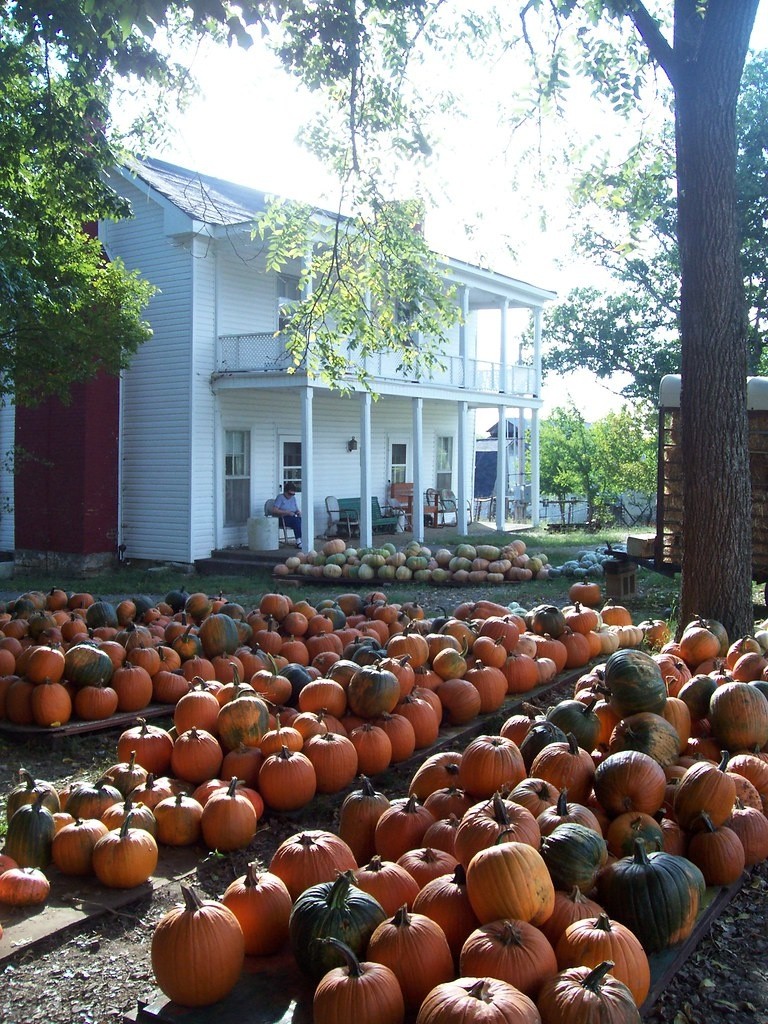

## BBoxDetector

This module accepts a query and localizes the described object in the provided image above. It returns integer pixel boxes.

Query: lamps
[347,436,358,452]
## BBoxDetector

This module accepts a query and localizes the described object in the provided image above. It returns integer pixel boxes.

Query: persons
[272,483,302,549]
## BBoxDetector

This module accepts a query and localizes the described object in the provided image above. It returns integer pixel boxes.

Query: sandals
[295,542,302,548]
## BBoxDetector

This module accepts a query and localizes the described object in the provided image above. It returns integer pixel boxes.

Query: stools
[246,518,280,551]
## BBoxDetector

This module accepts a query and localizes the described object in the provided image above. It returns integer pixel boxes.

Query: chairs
[440,489,473,527]
[379,506,413,532]
[426,488,458,530]
[264,499,301,549]
[325,496,359,541]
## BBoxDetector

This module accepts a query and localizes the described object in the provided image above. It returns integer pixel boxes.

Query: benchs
[391,482,439,532]
[336,496,398,536]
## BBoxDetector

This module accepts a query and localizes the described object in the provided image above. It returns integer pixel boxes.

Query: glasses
[286,491,295,496]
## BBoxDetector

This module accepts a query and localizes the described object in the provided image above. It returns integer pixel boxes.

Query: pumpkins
[0,536,768,1024]
